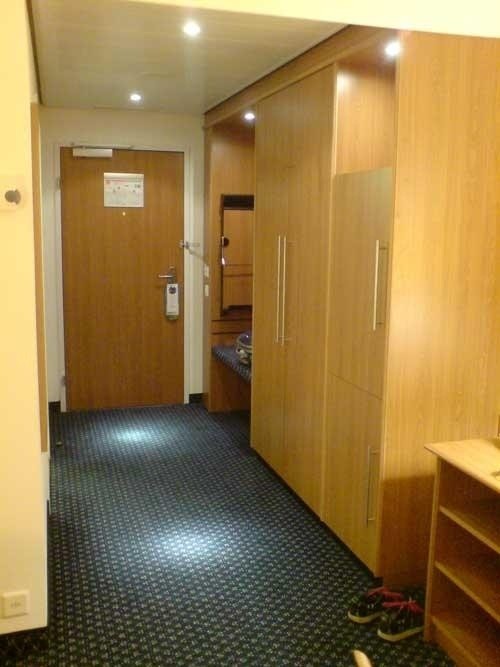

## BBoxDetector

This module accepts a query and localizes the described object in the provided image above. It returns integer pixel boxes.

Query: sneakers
[349,586,424,642]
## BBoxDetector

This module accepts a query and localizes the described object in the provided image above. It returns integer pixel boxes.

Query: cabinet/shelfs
[419,436,500,665]
[249,30,335,522]
[322,30,500,579]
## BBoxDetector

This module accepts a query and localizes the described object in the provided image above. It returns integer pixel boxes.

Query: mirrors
[220,193,253,316]
